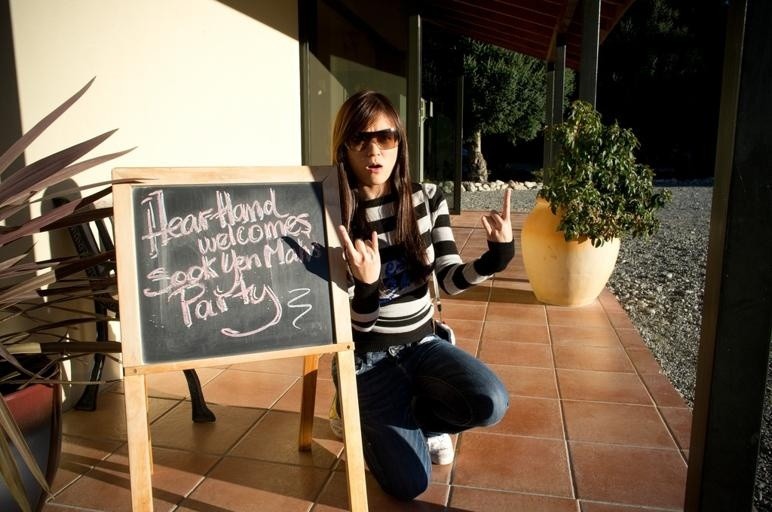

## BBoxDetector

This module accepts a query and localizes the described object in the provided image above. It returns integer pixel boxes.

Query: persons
[329,89,517,504]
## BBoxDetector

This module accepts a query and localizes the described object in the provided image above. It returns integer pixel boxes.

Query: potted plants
[523,100,673,310]
[2,70,159,512]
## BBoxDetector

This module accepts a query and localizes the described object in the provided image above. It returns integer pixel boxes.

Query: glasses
[341,128,400,152]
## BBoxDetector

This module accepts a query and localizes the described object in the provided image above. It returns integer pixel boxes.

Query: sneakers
[328,394,343,439]
[425,434,455,466]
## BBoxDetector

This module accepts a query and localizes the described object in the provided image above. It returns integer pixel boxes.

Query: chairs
[49,193,218,426]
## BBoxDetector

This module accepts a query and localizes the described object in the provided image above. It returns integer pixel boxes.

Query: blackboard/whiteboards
[112,165,357,375]
